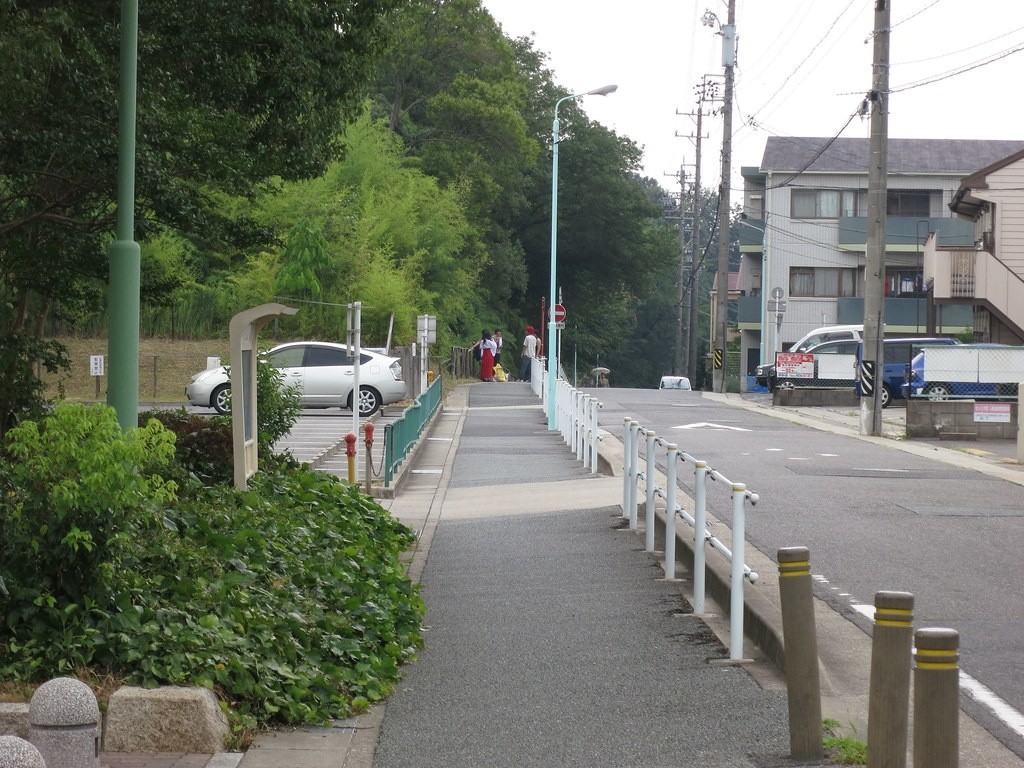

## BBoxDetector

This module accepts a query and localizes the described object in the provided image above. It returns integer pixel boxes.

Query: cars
[653,376,696,391]
[185,341,407,417]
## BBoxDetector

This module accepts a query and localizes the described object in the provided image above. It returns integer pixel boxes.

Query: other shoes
[516,379,523,383]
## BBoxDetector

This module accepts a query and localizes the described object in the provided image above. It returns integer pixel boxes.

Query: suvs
[754,323,1018,410]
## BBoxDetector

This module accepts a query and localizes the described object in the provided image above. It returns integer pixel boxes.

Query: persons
[474,326,542,382]
[599,373,610,388]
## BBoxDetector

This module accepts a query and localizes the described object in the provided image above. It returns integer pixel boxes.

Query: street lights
[548,85,619,431]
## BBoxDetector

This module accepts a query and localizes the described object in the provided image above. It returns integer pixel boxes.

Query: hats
[483,331,490,339]
[528,326,534,334]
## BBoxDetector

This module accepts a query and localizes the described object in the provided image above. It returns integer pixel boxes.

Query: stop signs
[547,303,566,322]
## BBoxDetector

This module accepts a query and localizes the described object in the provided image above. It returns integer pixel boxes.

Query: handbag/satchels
[474,347,481,361]
